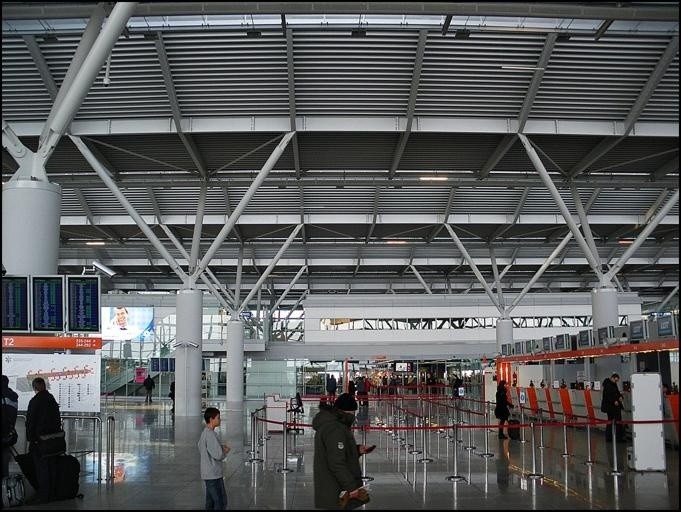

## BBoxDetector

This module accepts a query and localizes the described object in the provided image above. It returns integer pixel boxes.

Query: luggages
[39,455,81,502]
[7,446,38,508]
[508,406,520,440]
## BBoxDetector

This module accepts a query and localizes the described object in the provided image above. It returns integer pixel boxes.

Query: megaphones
[603,337,617,350]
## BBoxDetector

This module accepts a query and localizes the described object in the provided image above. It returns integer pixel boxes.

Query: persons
[312,393,368,510]
[494,381,514,439]
[143,375,155,402]
[105,306,138,340]
[296,392,304,414]
[0,376,18,476]
[326,374,463,407]
[601,374,627,443]
[198,407,231,510]
[27,377,62,454]
[170,382,175,411]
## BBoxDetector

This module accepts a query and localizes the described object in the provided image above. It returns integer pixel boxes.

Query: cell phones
[366,445,376,453]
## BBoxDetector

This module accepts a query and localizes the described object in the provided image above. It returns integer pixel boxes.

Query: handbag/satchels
[1,425,17,448]
[2,475,23,507]
[39,431,66,459]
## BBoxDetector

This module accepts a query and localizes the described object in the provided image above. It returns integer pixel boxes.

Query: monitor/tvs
[394,362,413,372]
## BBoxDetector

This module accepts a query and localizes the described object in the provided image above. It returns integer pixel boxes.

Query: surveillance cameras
[172,340,184,348]
[93,259,118,279]
[188,341,199,349]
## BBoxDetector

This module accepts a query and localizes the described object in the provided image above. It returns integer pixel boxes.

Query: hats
[334,393,357,411]
